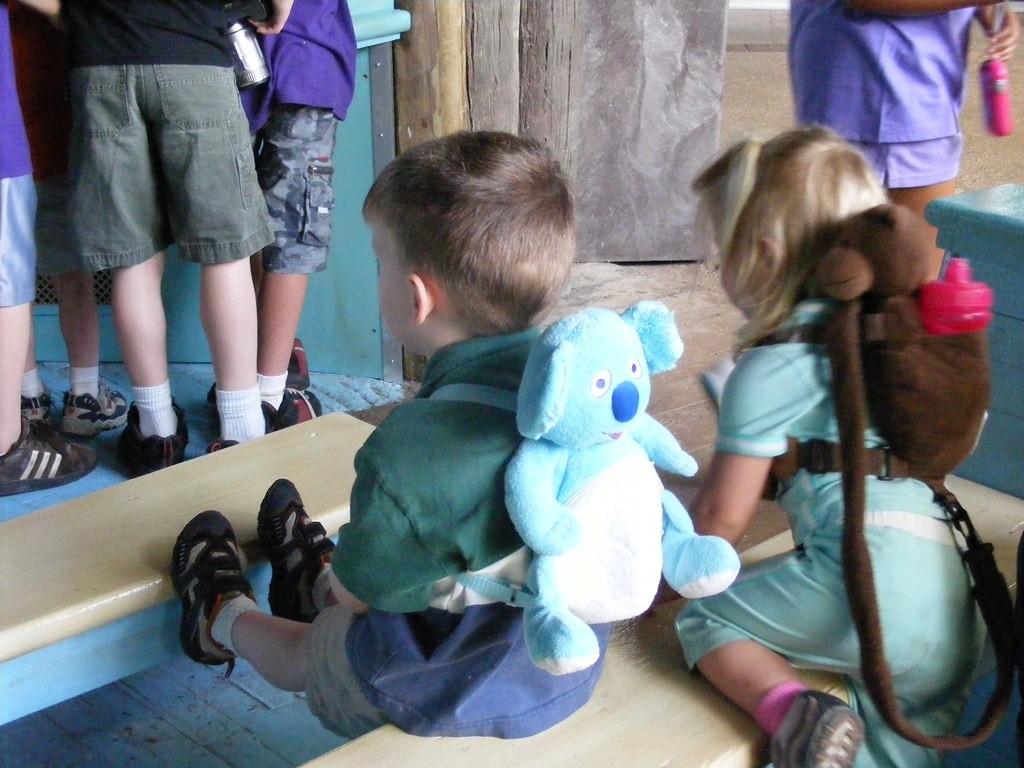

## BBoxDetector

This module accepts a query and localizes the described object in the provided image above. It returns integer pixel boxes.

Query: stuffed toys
[816,205,1015,749]
[506,298,741,674]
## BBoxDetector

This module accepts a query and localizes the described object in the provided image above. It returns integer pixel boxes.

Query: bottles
[981,58,1013,136]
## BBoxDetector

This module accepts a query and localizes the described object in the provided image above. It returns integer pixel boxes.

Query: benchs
[295,473,1024,768]
[923,183,1024,500]
[0,411,377,726]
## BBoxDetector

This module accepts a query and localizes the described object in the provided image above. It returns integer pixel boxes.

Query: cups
[225,19,270,88]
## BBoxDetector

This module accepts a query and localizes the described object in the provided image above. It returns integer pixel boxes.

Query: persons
[645,122,988,768]
[789,0,1020,218]
[0,0,295,497]
[170,131,606,741]
[207,0,360,434]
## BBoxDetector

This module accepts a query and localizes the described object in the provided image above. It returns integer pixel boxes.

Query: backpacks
[737,199,1017,748]
[427,300,741,674]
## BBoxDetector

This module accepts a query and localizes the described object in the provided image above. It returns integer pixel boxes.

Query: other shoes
[768,687,863,768]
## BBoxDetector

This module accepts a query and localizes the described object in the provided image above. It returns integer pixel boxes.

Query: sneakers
[59,376,128,435]
[0,433,98,496]
[171,511,256,678]
[284,338,312,390]
[261,386,322,439]
[256,480,339,623]
[20,389,56,440]
[117,395,188,478]
[208,401,283,460]
